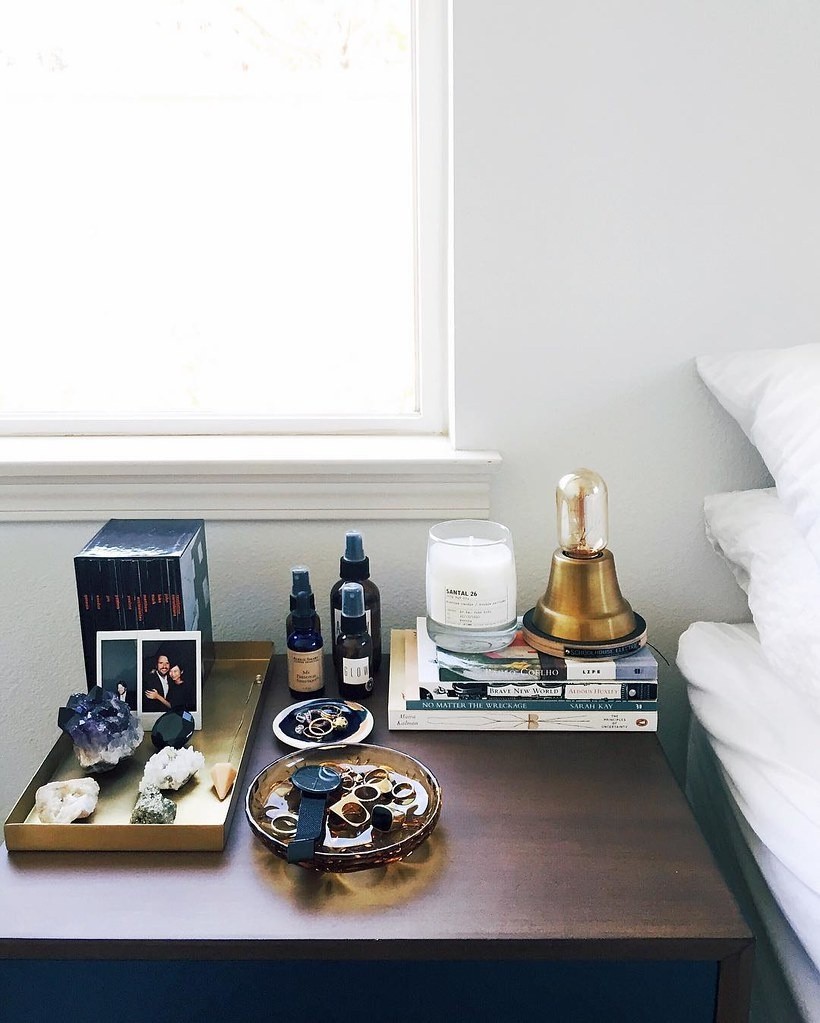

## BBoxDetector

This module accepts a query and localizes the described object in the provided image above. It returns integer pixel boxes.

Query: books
[384,615,659,732]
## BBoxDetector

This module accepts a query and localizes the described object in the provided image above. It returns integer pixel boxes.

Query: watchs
[287,765,341,862]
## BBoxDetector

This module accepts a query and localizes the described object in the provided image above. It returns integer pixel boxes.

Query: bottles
[285,564,321,639]
[335,583,374,701]
[287,591,325,700]
[329,531,382,675]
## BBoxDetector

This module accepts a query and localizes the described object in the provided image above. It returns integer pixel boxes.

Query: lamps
[521,469,647,664]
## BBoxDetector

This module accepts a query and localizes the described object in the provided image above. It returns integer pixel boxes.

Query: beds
[675,620,819,1023]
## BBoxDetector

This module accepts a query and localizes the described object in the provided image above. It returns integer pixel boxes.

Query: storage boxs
[73,518,217,697]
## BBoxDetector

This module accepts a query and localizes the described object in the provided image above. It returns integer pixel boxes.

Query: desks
[0,653,756,1023]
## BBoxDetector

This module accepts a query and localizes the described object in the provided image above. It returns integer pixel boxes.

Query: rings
[271,765,416,836]
[296,706,348,741]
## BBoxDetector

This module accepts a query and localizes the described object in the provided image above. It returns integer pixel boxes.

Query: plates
[272,697,374,749]
[244,742,443,874]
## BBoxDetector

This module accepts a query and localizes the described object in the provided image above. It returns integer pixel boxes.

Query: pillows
[703,486,819,700]
[696,342,819,565]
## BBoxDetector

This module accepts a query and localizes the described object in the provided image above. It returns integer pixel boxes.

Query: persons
[117,680,137,711]
[142,652,194,711]
